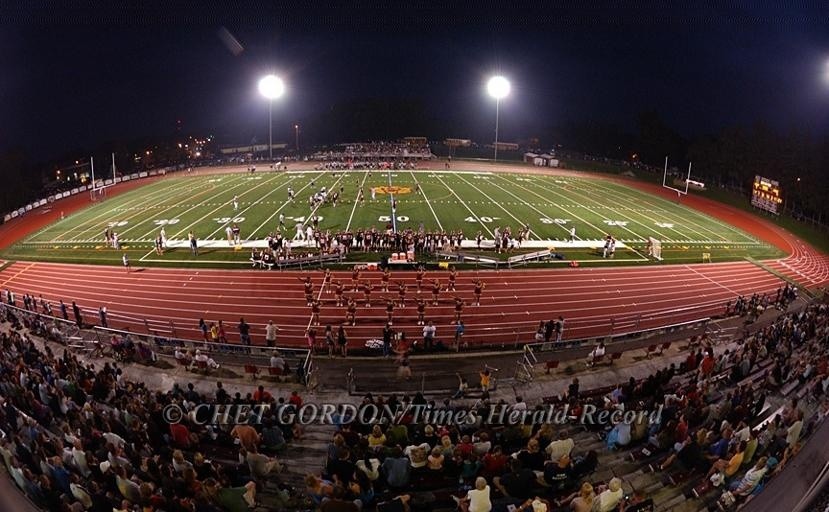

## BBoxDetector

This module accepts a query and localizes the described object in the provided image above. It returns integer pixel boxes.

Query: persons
[0,122,827,512]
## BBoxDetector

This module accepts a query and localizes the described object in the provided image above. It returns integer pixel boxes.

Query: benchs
[0,281,829,512]
